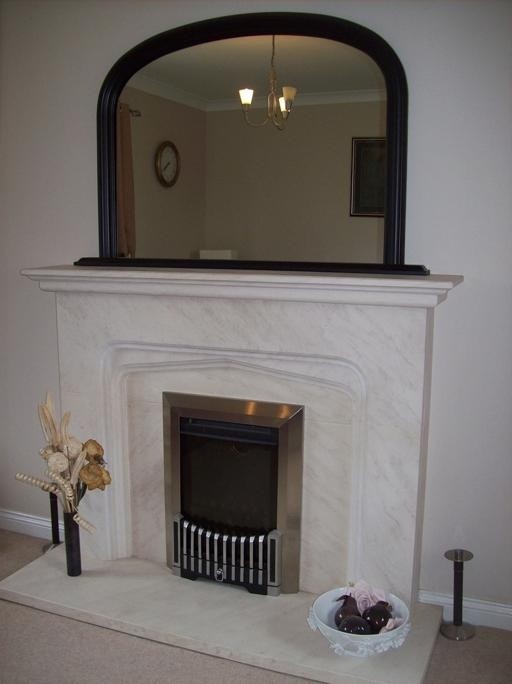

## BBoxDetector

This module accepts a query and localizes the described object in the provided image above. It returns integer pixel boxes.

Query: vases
[63,510,82,577]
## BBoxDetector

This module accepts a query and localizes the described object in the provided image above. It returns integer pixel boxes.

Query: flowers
[16,392,114,535]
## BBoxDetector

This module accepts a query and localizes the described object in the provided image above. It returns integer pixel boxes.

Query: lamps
[237,33,298,133]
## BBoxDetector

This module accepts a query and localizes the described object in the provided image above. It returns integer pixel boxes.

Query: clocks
[156,140,181,188]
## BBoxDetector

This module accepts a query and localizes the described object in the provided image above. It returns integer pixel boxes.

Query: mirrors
[95,10,409,266]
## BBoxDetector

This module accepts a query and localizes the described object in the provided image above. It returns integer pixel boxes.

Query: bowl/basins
[311,587,410,652]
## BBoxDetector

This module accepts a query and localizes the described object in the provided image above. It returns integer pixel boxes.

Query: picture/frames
[348,136,389,218]
